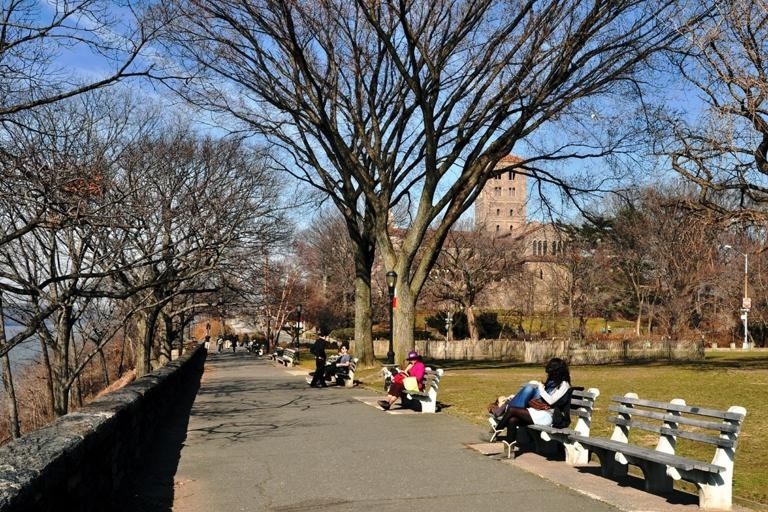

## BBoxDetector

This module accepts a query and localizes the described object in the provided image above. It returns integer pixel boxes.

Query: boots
[493,440,516,460]
[479,425,504,443]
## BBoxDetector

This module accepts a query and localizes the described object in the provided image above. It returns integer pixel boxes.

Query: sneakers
[377,400,390,409]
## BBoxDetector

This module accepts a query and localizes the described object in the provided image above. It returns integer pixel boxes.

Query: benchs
[399,366,446,415]
[519,386,750,508]
[268,346,298,369]
[325,353,360,389]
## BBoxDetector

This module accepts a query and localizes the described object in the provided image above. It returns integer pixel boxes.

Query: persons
[203,331,268,358]
[324,340,351,381]
[309,331,328,389]
[479,356,575,462]
[376,351,425,409]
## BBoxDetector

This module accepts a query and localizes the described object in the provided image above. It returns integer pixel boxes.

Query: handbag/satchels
[394,371,419,392]
[509,384,551,410]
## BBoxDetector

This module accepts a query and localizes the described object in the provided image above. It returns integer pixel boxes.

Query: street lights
[385,271,399,364]
[295,303,303,348]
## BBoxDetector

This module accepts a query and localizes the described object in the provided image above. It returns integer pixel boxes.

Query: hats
[406,352,421,360]
